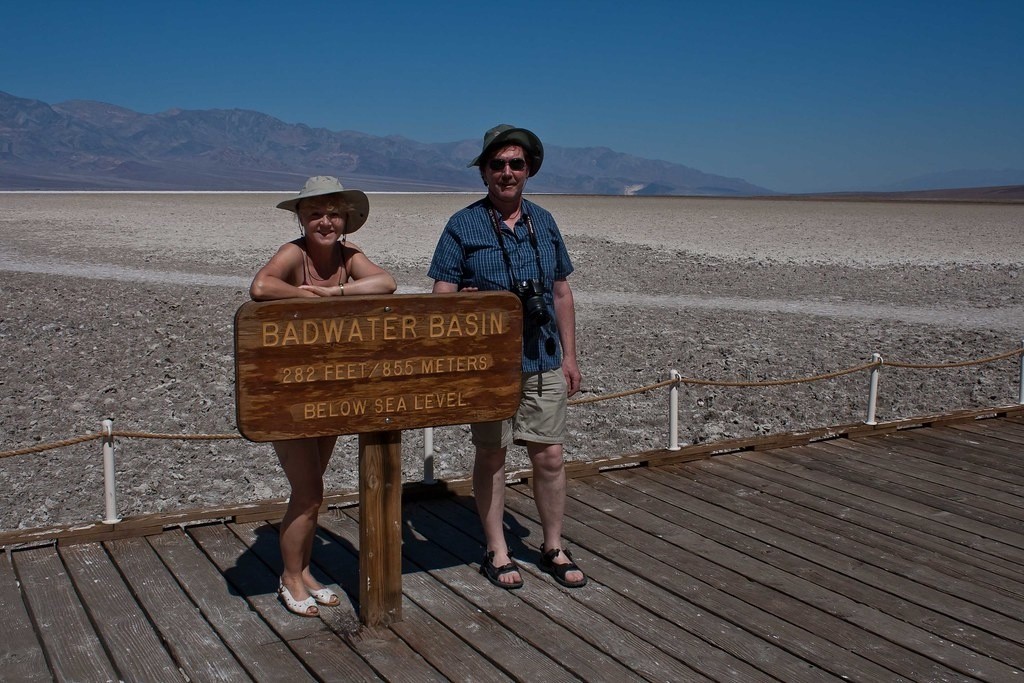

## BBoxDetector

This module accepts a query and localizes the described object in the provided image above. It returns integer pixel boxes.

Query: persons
[427,123,587,589]
[249,175,398,618]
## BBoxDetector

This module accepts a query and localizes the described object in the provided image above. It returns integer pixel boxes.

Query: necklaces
[493,209,521,219]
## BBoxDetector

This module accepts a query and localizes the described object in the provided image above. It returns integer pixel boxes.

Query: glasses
[489,158,527,170]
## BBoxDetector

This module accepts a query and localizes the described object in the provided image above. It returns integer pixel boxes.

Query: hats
[275,175,370,235]
[466,124,544,177]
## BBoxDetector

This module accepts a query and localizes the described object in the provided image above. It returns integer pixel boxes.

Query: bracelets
[339,282,345,295]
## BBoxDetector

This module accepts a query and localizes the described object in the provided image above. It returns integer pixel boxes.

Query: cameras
[512,278,550,327]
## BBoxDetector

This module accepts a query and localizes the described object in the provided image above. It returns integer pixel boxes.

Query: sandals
[304,577,341,607]
[277,575,320,617]
[479,544,524,588]
[536,542,588,587]
